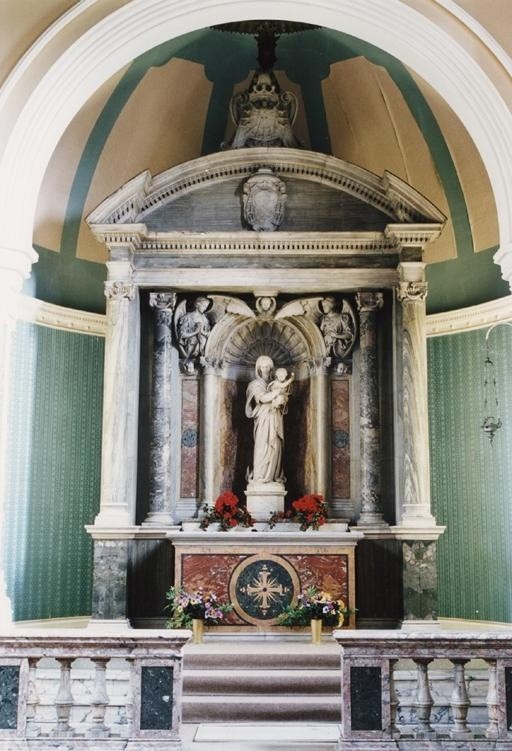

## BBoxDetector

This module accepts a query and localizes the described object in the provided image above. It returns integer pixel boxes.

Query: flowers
[276,584,359,630]
[160,583,234,630]
[195,489,256,534]
[263,492,329,532]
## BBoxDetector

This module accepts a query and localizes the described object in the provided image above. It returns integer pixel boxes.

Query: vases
[190,618,204,644]
[310,618,322,644]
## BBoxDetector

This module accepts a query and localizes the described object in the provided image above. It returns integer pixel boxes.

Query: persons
[177,296,212,373]
[319,296,353,367]
[266,367,295,409]
[244,355,290,483]
